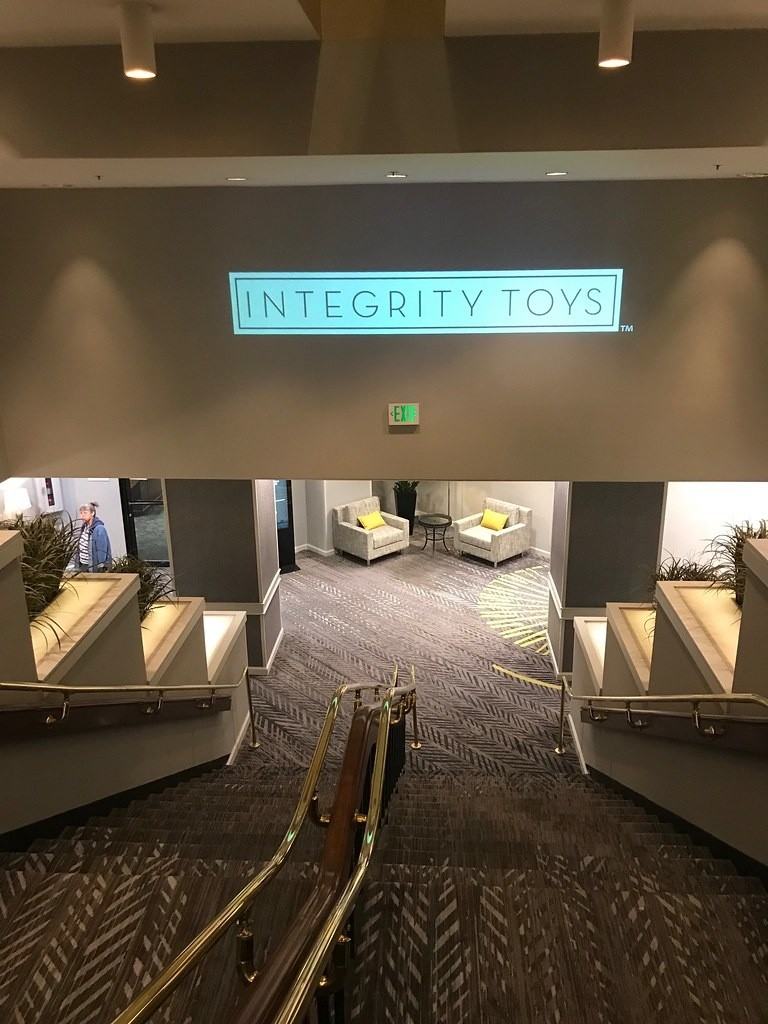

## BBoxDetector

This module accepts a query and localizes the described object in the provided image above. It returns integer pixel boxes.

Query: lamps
[118,2,157,79]
[598,1,636,68]
[4,488,32,526]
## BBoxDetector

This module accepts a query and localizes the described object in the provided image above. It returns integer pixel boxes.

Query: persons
[73,502,111,572]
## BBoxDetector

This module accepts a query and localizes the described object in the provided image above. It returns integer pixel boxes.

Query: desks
[418,513,452,558]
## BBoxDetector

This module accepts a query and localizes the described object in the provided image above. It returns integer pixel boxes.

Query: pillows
[357,511,387,530]
[481,509,509,531]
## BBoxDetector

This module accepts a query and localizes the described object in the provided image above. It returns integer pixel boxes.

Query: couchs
[332,496,410,566]
[453,498,532,567]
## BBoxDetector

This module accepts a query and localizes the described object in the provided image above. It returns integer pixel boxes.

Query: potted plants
[93,548,180,630]
[393,481,420,535]
[701,519,768,626]
[0,507,109,652]
